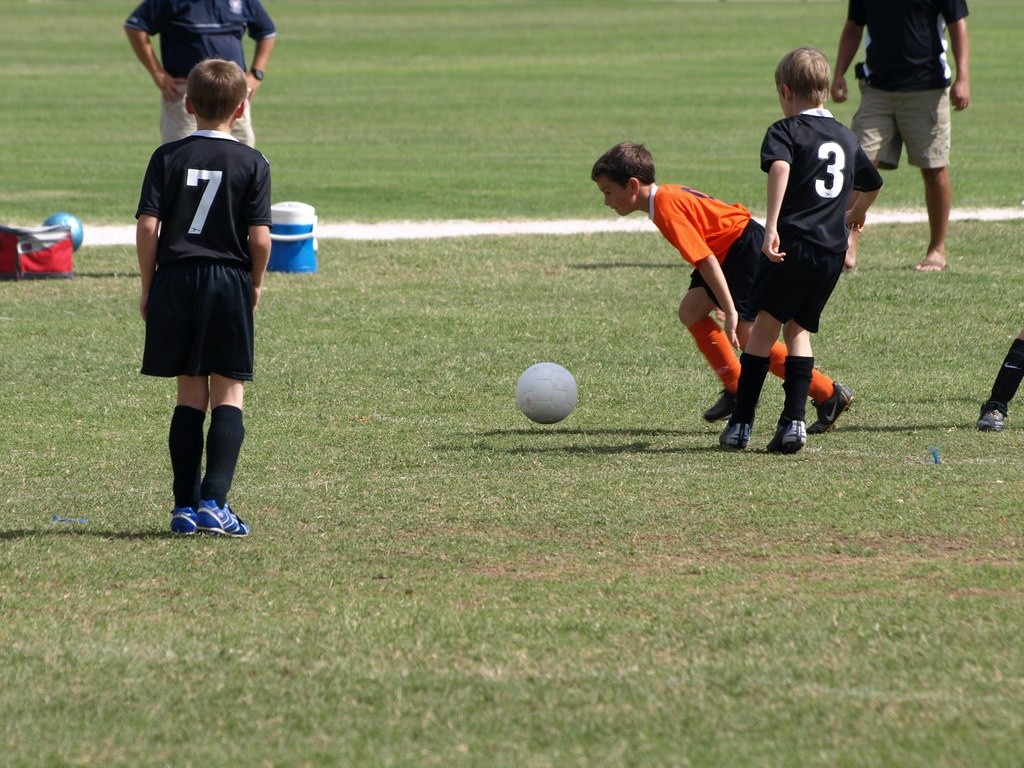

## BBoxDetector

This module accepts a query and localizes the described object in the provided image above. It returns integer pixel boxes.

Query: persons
[976,329,1024,432]
[124,0,277,146]
[136,59,271,537]
[721,45,883,450]
[592,141,855,435]
[832,0,970,271]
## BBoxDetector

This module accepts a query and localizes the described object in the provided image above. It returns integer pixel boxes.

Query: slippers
[917,260,947,272]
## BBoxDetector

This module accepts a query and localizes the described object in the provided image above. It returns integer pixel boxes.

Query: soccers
[42,211,84,253]
[515,362,578,424]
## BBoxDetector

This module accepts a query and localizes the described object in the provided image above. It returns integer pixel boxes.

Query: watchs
[250,68,264,80]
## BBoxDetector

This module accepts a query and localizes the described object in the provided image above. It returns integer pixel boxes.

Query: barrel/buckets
[264,200,317,274]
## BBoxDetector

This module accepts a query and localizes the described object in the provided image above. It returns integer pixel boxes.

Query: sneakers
[169,506,199,534]
[198,500,249,537]
[766,417,807,453]
[719,422,755,449]
[703,389,761,424]
[976,408,1007,431]
[806,381,855,434]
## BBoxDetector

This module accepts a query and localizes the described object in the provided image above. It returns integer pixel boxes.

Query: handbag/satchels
[0,224,74,280]
